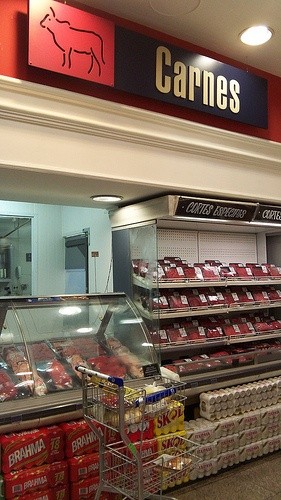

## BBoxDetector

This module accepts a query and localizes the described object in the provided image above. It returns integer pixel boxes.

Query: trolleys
[75,363,222,499]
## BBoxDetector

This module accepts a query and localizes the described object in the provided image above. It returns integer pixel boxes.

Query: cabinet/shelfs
[110,195,280,405]
